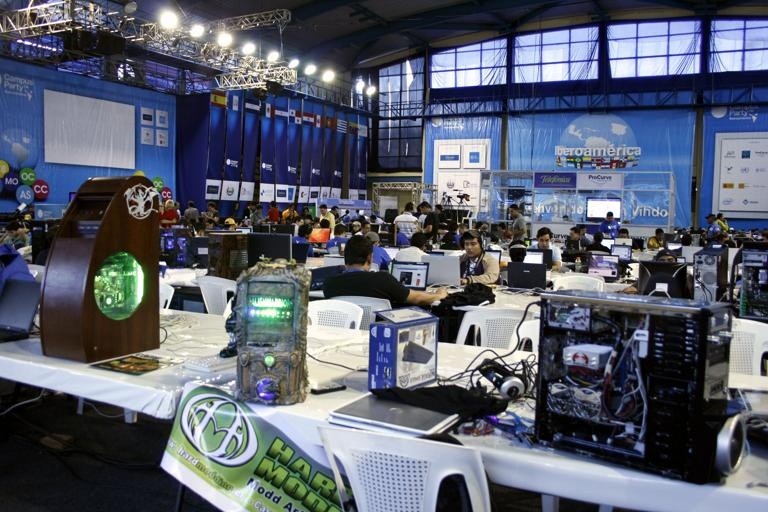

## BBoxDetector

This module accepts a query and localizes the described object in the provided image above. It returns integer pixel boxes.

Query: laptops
[327,392,460,436]
[614,238,633,245]
[525,251,544,265]
[421,255,465,288]
[507,261,547,288]
[527,248,552,269]
[586,253,619,283]
[611,244,632,260]
[390,260,430,291]
[0,278,41,342]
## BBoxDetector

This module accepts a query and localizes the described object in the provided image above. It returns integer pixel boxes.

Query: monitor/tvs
[638,261,688,298]
[308,228,331,248]
[585,196,622,223]
[235,228,251,233]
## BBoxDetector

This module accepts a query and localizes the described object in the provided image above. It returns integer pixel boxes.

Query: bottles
[524,238,530,248]
[575,257,582,272]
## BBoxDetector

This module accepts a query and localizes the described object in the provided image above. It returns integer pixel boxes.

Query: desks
[165,257,346,315]
[0,309,370,421]
[429,286,542,314]
[172,340,768,512]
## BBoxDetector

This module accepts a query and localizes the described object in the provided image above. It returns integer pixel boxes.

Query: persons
[4,222,33,250]
[159,198,730,309]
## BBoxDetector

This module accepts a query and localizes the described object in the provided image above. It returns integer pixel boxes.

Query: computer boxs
[693,247,728,300]
[739,240,768,322]
[533,289,730,484]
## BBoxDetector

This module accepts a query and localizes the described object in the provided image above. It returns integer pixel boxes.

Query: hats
[463,230,480,240]
[656,250,677,262]
[225,218,237,227]
[365,232,380,241]
[705,214,715,219]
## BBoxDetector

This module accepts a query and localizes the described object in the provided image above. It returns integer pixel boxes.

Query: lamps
[123,1,137,13]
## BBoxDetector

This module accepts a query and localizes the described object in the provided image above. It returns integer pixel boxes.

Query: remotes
[311,382,346,394]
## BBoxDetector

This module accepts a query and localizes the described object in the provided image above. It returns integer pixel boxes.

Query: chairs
[316,425,490,512]
[456,307,534,350]
[726,317,768,392]
[307,299,363,330]
[489,318,613,511]
[76,282,175,425]
[553,276,603,292]
[198,276,237,315]
[330,295,393,330]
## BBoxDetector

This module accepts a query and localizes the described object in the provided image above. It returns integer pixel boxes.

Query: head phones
[479,358,531,401]
